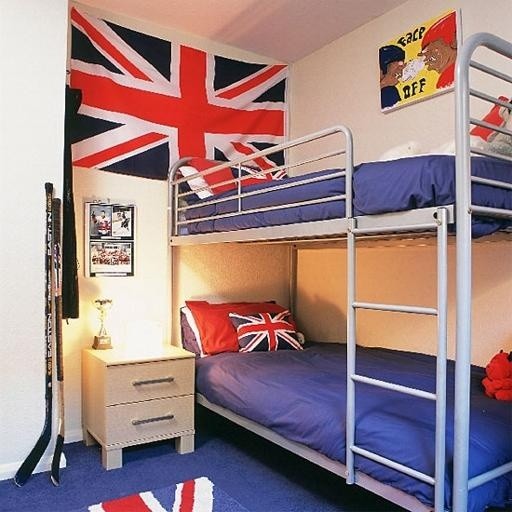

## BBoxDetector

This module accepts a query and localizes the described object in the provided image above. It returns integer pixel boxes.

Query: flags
[71,6,288,184]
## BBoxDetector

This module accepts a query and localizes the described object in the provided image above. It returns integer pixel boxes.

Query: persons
[91,209,132,266]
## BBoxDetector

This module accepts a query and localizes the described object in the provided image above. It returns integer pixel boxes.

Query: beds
[168,32,511,511]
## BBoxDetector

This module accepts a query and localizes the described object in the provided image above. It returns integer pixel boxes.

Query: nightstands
[82,343,196,472]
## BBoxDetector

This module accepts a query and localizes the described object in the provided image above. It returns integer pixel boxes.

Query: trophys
[91,294,114,351]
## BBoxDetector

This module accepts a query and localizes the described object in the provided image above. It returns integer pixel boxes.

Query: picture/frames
[85,201,138,278]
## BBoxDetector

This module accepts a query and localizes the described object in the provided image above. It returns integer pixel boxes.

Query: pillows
[180,300,304,358]
[176,157,289,208]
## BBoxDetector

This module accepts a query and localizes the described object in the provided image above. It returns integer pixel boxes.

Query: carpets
[72,476,247,512]
